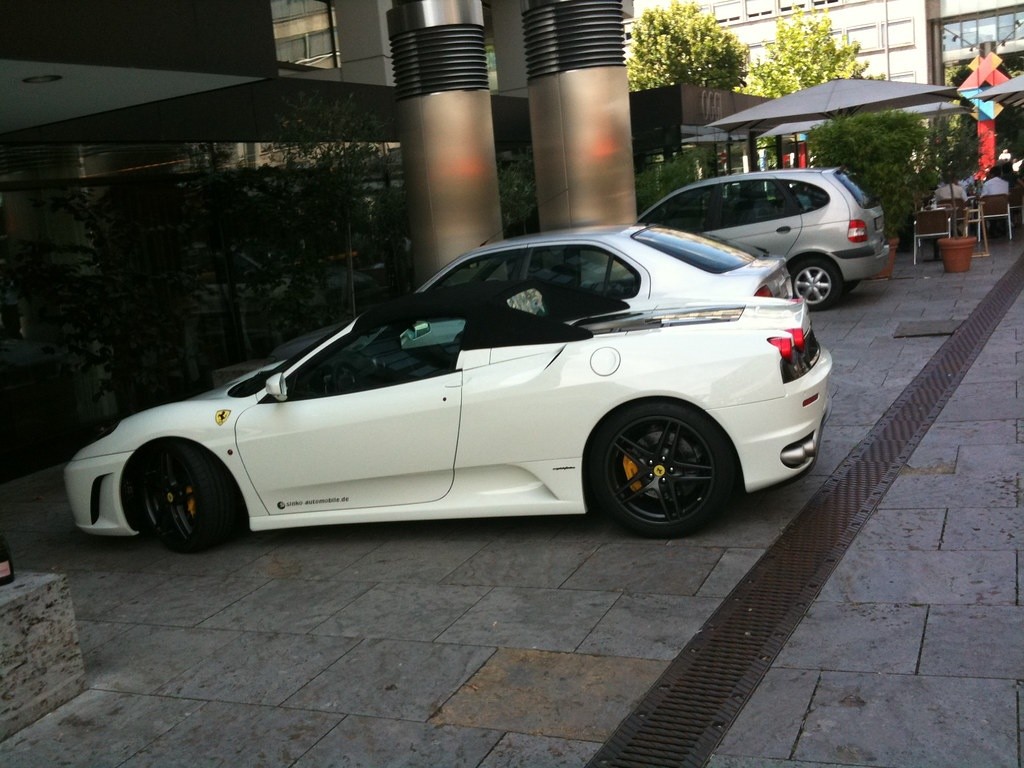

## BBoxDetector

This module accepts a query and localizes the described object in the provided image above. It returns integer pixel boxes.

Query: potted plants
[805,105,946,280]
[937,113,984,273]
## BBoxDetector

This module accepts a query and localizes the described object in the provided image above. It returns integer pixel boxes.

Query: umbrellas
[704,73,1024,140]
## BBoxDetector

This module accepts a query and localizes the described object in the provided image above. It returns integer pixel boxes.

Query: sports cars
[63,291,832,554]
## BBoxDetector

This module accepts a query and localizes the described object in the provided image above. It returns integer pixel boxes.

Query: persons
[933,162,1024,237]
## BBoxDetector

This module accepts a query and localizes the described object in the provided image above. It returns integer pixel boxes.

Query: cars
[264,167,890,367]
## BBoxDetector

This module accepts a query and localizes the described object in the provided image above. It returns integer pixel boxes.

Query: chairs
[978,194,1013,242]
[913,207,951,265]
[936,198,969,237]
[551,263,577,288]
[1009,186,1024,223]
